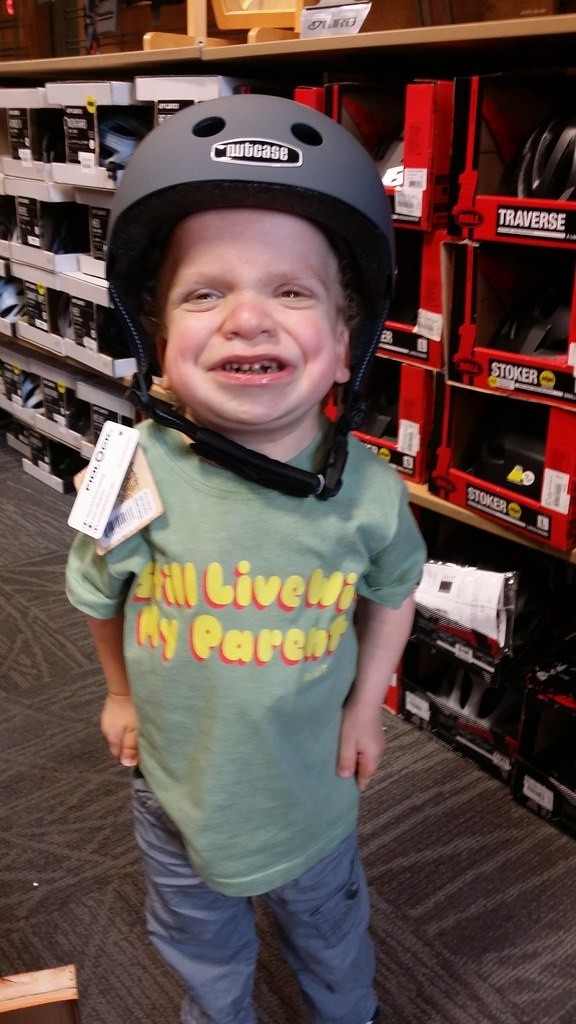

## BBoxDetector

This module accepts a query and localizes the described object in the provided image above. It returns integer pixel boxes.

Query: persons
[66,95,425,1024]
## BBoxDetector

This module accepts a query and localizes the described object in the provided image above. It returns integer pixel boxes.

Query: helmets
[0,278,27,320]
[105,94,395,393]
[491,284,569,355]
[99,116,148,172]
[22,377,45,414]
[517,118,575,201]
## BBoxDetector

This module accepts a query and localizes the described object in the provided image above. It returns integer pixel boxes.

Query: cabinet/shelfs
[0,0,576,566]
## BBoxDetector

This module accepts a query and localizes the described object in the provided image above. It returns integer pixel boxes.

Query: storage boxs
[0,74,576,843]
